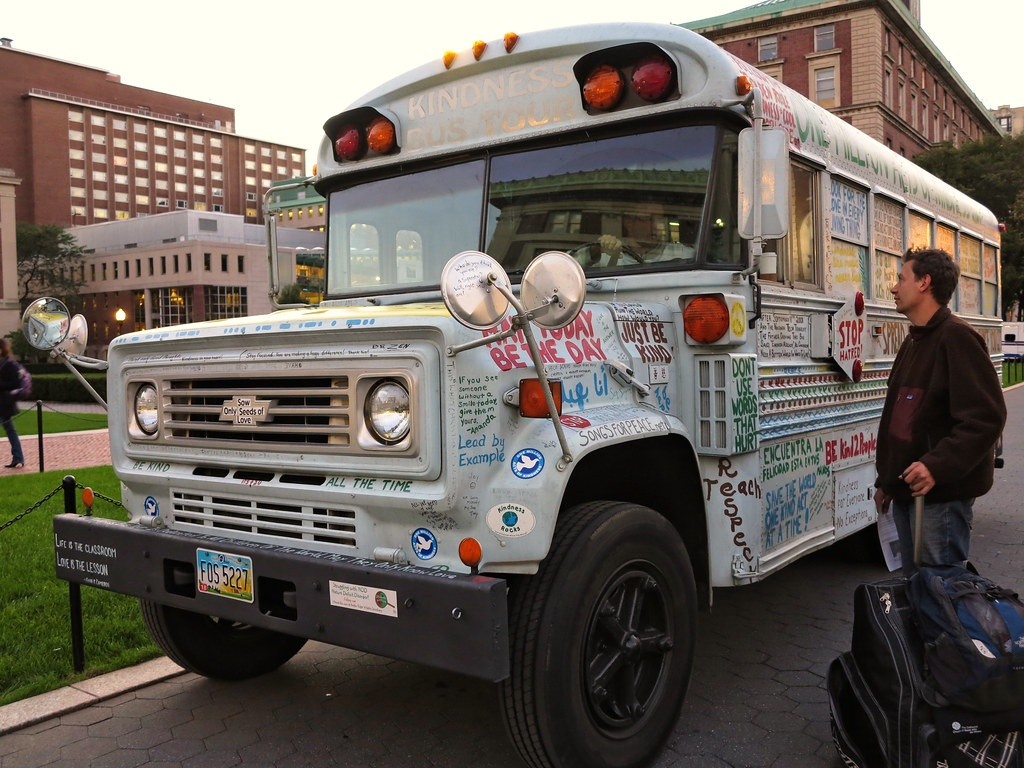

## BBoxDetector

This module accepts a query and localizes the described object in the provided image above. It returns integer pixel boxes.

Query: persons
[565,191,696,269]
[872,246,1008,578]
[0,337,26,468]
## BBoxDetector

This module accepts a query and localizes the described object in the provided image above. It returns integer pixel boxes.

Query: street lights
[114,308,126,337]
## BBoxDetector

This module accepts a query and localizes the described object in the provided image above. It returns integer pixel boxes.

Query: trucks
[54,20,1007,768]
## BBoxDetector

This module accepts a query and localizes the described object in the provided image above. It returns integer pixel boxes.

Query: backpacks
[6,362,34,399]
[907,560,1023,712]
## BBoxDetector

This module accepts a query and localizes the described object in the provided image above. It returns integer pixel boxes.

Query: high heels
[5,461,25,469]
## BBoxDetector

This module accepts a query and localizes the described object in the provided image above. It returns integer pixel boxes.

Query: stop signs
[832,290,868,384]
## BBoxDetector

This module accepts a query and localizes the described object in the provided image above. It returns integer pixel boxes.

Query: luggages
[824,577,1022,768]
